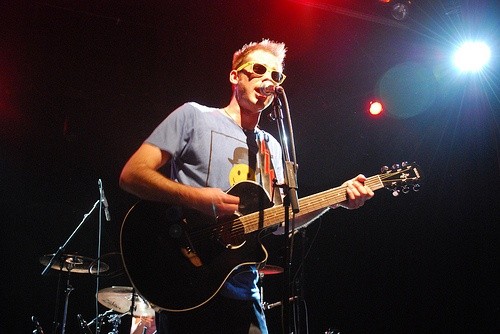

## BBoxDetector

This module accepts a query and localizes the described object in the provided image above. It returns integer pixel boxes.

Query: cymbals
[38,252,110,274]
[96,286,161,316]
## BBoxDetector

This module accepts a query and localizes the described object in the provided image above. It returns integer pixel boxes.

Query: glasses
[236,60,287,85]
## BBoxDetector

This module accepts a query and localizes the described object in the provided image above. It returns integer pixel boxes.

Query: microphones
[36,321,45,334]
[98,180,111,221]
[259,80,283,95]
[80,320,92,334]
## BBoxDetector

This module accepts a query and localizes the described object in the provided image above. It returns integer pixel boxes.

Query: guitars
[119,158,425,312]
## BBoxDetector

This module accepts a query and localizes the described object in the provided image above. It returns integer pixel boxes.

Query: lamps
[391,0,414,20]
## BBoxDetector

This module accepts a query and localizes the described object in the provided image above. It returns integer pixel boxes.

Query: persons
[118,39,374,334]
